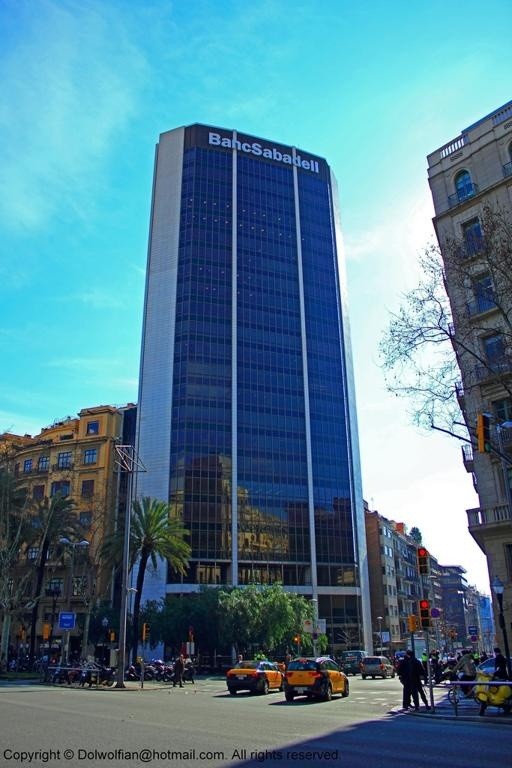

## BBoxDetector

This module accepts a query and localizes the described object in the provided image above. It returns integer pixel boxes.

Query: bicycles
[448,671,482,705]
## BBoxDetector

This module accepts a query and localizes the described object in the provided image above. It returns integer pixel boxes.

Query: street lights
[377,616,383,655]
[491,574,511,679]
[59,538,89,612]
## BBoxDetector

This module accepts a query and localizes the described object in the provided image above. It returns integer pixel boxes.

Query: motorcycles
[51,657,196,686]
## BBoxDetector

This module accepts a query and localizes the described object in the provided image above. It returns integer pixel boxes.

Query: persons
[236,655,242,664]
[398,650,414,710]
[421,646,489,684]
[403,651,431,711]
[172,654,186,687]
[492,648,508,680]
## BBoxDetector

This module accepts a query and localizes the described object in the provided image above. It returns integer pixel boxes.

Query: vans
[342,651,370,676]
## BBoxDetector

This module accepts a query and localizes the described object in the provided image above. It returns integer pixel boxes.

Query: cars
[360,655,395,678]
[34,655,51,674]
[476,658,499,673]
[226,656,349,702]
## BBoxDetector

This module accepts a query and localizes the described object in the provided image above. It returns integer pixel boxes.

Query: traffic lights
[107,630,117,645]
[143,623,151,641]
[418,600,431,630]
[416,546,430,575]
